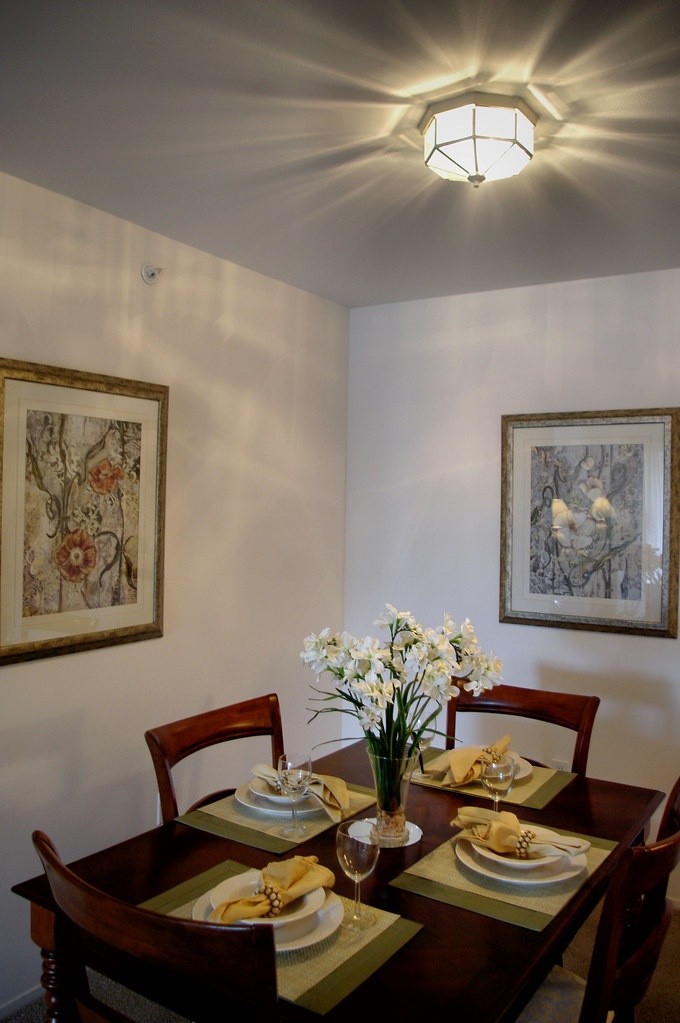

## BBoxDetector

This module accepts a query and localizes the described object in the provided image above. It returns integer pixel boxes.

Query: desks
[11,739,668,1023]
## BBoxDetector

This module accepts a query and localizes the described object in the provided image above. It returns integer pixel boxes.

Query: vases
[365,743,421,839]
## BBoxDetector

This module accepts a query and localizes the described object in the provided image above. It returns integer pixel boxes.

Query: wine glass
[336,820,379,931]
[277,753,312,839]
[481,755,514,812]
[410,712,436,780]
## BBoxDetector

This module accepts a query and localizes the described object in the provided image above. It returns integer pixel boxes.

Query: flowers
[300,603,503,810]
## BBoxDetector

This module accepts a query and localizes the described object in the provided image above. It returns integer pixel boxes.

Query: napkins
[253,762,350,823]
[209,855,335,924]
[450,805,592,856]
[424,735,511,787]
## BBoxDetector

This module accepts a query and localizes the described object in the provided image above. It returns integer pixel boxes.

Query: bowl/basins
[493,750,520,768]
[471,824,564,869]
[249,778,313,804]
[210,870,325,925]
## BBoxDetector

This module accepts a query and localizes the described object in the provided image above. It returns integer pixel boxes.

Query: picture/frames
[0,358,169,666]
[499,407,680,639]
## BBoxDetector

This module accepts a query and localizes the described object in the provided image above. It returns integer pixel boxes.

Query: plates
[193,888,343,951]
[455,839,587,884]
[234,784,324,817]
[515,756,533,784]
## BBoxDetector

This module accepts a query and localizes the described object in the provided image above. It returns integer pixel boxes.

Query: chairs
[32,829,279,1023]
[446,676,600,778]
[144,692,288,825]
[514,775,680,1023]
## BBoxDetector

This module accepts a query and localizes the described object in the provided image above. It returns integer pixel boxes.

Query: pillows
[418,91,540,189]
